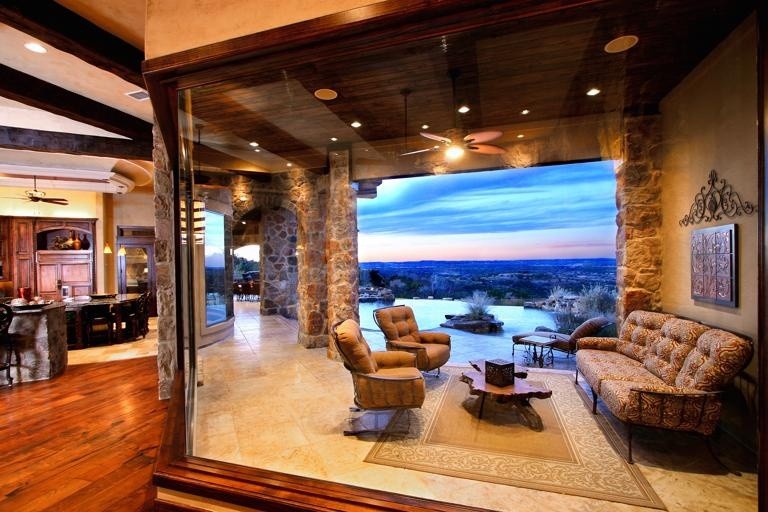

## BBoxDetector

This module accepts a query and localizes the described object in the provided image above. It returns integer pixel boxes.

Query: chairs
[0,303,20,388]
[512,317,614,359]
[373,305,451,377]
[65,291,152,350]
[330,320,425,436]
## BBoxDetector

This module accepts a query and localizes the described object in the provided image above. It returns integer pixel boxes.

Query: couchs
[575,310,754,464]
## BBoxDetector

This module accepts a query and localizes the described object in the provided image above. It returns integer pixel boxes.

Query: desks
[519,336,559,368]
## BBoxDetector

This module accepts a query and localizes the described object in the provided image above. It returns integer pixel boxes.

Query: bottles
[54,230,81,250]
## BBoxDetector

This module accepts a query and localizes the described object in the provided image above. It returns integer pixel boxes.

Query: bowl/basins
[10,296,45,306]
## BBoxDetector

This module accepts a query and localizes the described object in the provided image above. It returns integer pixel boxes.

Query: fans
[401,68,507,155]
[0,176,68,205]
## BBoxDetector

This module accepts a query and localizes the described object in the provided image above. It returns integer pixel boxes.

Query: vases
[73,230,81,250]
[68,231,74,247]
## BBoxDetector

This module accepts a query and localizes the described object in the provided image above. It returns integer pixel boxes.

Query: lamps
[104,242,112,254]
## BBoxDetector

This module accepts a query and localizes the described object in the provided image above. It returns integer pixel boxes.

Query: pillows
[615,310,752,392]
[334,319,378,375]
[568,317,607,345]
[376,306,422,343]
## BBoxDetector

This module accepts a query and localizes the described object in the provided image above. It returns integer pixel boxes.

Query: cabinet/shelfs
[36,250,93,295]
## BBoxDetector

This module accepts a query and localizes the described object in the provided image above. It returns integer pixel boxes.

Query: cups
[18,286,31,302]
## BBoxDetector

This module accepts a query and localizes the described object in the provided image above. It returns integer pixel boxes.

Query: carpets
[363,366,669,511]
